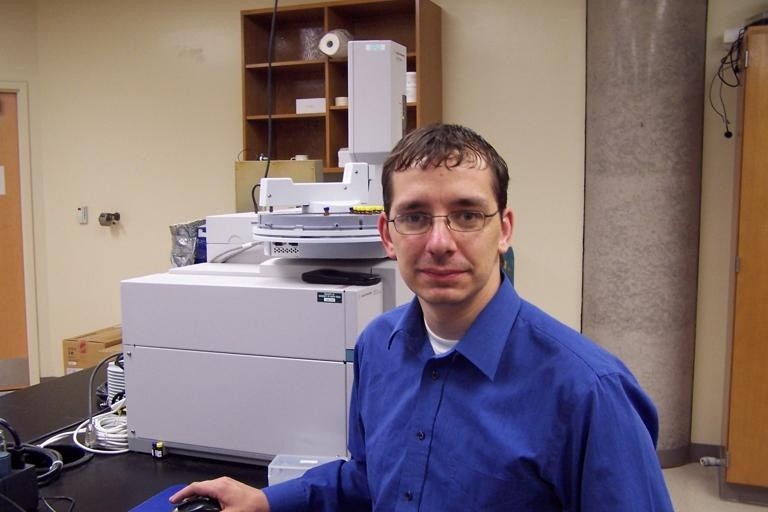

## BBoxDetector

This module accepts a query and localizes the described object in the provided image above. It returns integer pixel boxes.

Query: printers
[205,208,302,264]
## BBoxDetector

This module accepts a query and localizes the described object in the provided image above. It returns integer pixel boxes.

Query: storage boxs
[63,327,124,375]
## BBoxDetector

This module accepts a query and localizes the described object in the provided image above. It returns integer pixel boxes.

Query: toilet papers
[317,29,354,60]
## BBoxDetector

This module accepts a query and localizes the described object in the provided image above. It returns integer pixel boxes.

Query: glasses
[384,210,500,235]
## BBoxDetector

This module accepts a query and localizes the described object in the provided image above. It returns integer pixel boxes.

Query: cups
[291,154,308,161]
[335,96,347,106]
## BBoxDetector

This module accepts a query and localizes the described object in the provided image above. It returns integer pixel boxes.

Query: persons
[167,123,676,512]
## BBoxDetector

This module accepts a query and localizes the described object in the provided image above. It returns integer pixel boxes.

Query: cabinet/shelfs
[237,1,444,174]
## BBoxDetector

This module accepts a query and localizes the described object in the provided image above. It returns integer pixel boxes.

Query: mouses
[171,498,222,512]
